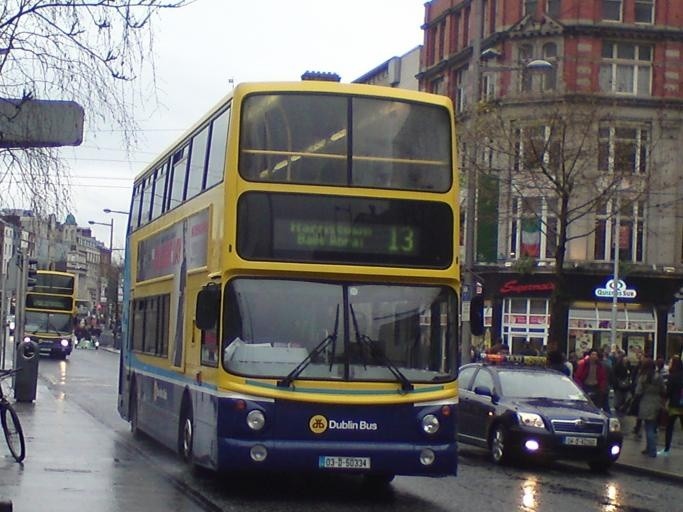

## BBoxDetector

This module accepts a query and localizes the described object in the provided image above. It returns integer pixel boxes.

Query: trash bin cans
[14,340,40,403]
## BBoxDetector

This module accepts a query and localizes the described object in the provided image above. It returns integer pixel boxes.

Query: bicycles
[0,368,29,461]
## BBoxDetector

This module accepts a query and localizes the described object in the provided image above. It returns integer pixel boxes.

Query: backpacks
[673,388,683,407]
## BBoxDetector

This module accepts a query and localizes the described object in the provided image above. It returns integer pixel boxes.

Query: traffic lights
[28,256,37,288]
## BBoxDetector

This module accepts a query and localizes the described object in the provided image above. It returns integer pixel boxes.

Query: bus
[118,71,484,478]
[23,270,77,359]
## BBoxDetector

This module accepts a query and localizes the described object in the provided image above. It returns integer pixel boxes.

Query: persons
[72,311,122,348]
[562,345,683,455]
[470,337,546,363]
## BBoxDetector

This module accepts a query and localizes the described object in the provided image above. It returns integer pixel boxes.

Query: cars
[458,353,624,472]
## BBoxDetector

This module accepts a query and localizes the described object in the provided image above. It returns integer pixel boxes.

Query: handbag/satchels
[618,381,631,392]
[622,398,639,416]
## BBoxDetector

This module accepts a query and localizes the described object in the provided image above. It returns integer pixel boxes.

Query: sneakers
[641,449,657,457]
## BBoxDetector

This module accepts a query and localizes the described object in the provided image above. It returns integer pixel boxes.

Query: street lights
[88,218,114,264]
[104,208,129,214]
[464,47,554,362]
